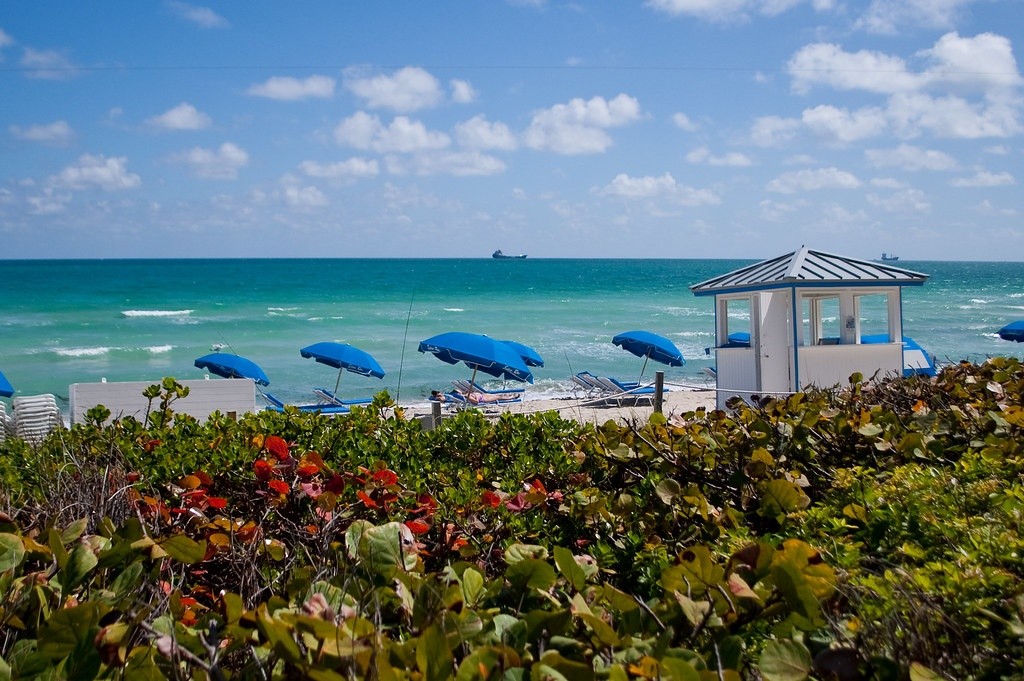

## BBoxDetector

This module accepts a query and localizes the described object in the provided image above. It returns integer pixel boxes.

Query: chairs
[0,369,670,444]
[701,366,716,387]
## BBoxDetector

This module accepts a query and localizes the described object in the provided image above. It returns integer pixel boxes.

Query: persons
[452,389,520,403]
[432,390,447,403]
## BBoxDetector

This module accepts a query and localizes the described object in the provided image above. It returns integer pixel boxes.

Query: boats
[881,253,899,260]
[492,250,527,259]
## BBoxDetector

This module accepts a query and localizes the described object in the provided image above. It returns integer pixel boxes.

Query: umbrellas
[195,353,270,387]
[729,332,750,343]
[417,332,534,414]
[301,341,385,403]
[499,340,544,390]
[0,371,15,398]
[999,320,1024,343]
[612,330,686,385]
